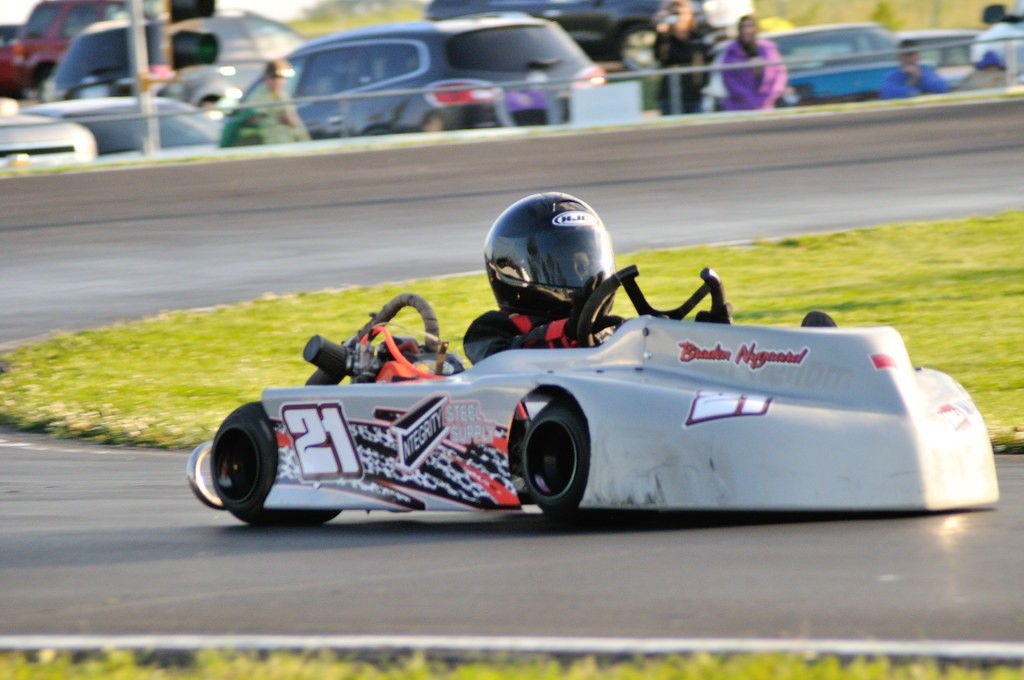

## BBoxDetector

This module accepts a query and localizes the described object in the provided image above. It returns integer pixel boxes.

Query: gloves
[571,271,622,341]
[695,302,734,324]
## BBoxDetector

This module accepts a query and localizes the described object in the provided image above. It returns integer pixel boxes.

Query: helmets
[484,192,616,323]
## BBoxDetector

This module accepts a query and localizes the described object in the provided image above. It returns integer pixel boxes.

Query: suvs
[215,7,606,147]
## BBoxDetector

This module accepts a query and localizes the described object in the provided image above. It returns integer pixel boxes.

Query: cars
[0,0,250,163]
[424,0,729,61]
[696,21,911,114]
[898,27,983,89]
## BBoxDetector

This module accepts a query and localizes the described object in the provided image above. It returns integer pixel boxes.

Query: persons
[226,61,310,145]
[463,191,625,366]
[719,14,787,111]
[653,0,718,114]
[879,40,949,100]
